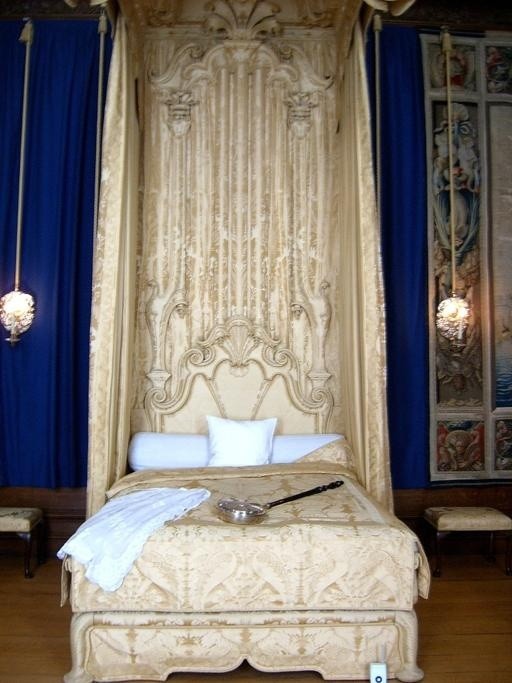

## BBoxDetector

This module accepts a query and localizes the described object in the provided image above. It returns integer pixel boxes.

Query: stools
[424,506,512,577]
[0,506,47,578]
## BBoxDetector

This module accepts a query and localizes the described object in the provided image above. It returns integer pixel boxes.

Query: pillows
[272,433,347,464]
[126,432,209,470]
[205,415,277,465]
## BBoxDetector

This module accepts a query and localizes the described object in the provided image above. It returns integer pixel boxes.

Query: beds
[57,317,434,683]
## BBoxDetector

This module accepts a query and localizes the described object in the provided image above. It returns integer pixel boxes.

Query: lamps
[437,26,474,343]
[0,17,34,347]
